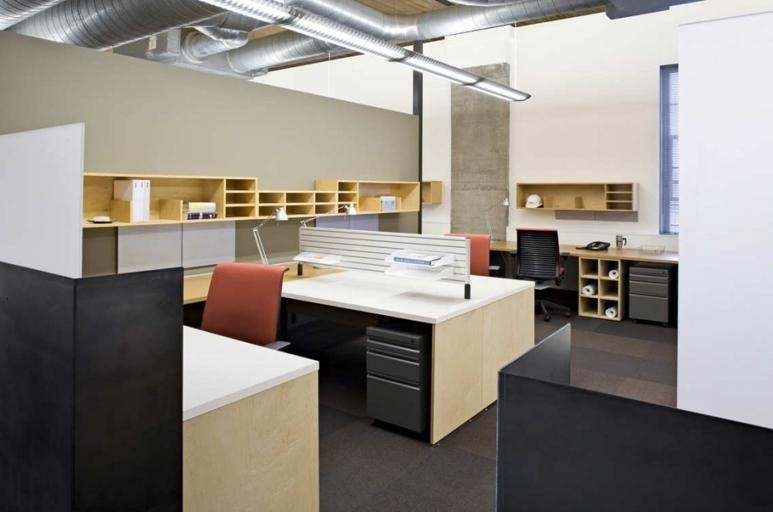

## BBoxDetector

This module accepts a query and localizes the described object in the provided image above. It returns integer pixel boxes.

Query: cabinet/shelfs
[445,235,678,326]
[83,173,443,310]
[515,182,639,214]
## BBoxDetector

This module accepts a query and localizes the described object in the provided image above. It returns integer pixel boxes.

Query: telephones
[586,241,610,250]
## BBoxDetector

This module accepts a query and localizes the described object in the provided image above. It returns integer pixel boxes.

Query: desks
[183,256,536,512]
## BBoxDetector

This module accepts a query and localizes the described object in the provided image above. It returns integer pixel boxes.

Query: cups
[615,235,626,250]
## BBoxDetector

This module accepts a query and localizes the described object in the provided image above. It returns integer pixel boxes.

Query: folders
[394,253,445,265]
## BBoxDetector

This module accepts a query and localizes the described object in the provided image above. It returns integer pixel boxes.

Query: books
[183,201,216,211]
[392,249,444,266]
[184,212,218,219]
[293,251,340,265]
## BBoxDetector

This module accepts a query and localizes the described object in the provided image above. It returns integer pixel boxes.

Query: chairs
[446,233,490,277]
[513,226,564,323]
[200,261,293,352]
